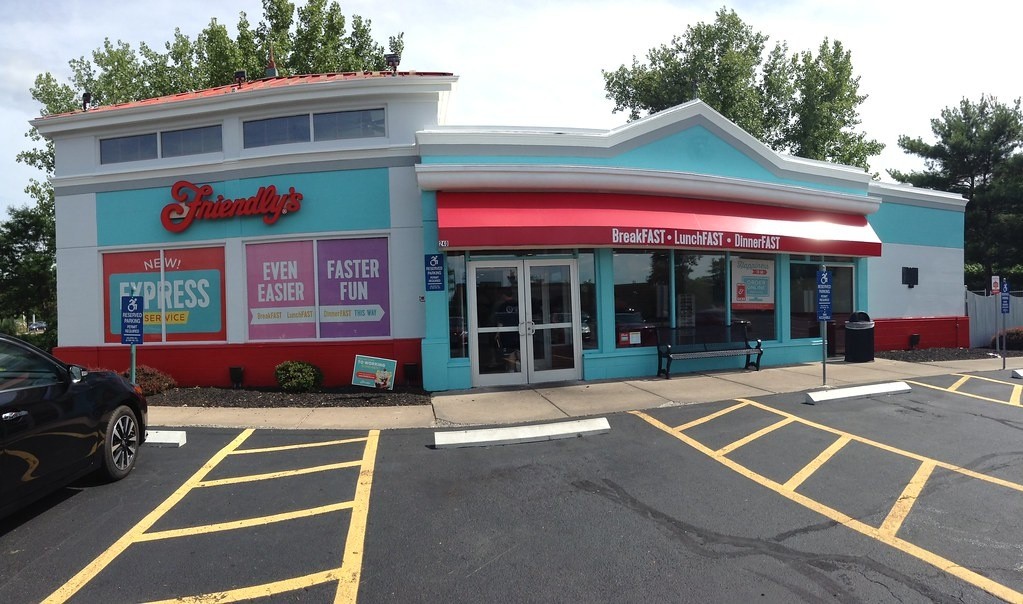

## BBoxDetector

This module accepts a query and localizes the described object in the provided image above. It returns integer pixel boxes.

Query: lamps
[385,54,400,76]
[235,70,247,89]
[82,93,91,111]
[228,365,244,388]
[908,334,921,347]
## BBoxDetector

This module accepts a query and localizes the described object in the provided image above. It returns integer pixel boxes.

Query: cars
[29,321,47,332]
[494,319,557,351]
[551,313,591,338]
[0,332,149,518]
[696,309,751,340]
[615,313,656,338]
[449,317,469,346]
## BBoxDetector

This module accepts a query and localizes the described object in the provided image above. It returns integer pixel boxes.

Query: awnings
[435,189,882,256]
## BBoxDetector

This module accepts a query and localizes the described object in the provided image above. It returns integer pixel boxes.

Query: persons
[495,288,520,373]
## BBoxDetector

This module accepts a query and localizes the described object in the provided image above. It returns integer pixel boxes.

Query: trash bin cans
[844,310,875,362]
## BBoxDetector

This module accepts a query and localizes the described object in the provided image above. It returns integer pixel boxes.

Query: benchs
[654,320,764,380]
